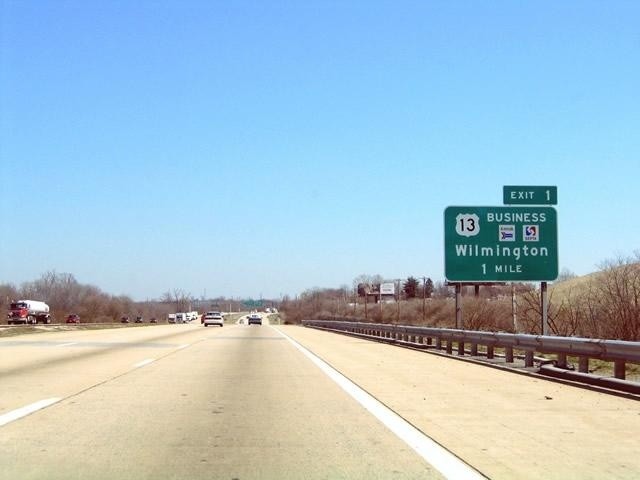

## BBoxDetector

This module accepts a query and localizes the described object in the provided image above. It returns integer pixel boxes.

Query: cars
[149,318,159,323]
[247,314,263,325]
[65,314,82,323]
[120,316,131,323]
[201,312,224,327]
[135,317,144,323]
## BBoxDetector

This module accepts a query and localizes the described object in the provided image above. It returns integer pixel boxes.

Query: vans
[168,311,198,323]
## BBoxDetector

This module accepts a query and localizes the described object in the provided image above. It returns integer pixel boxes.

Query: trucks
[7,299,52,324]
